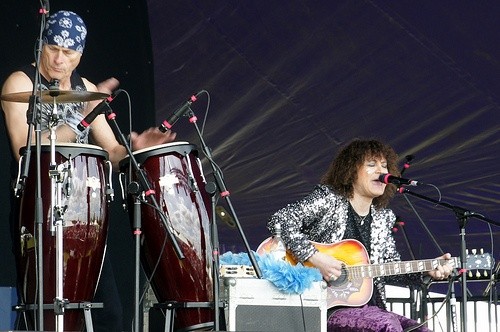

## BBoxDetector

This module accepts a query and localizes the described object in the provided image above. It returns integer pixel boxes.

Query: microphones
[159,90,205,134]
[378,173,423,186]
[77,89,123,132]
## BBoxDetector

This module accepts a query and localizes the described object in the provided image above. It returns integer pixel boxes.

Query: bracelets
[64,111,89,144]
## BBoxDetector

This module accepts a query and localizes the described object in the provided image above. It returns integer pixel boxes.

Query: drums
[120,142,220,332]
[18,143,109,332]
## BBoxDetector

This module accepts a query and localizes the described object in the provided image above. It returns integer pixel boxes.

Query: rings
[330,275,335,281]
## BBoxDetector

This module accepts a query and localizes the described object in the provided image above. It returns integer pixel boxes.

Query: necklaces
[352,200,368,225]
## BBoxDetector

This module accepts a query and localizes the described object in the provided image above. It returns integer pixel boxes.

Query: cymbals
[0,91,111,103]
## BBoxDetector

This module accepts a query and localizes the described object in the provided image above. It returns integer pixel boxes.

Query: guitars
[255,235,494,311]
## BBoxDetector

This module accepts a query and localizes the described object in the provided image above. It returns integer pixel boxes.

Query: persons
[266,139,451,332]
[0,10,177,285]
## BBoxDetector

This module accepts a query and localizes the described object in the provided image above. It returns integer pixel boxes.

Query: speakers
[226,278,328,332]
